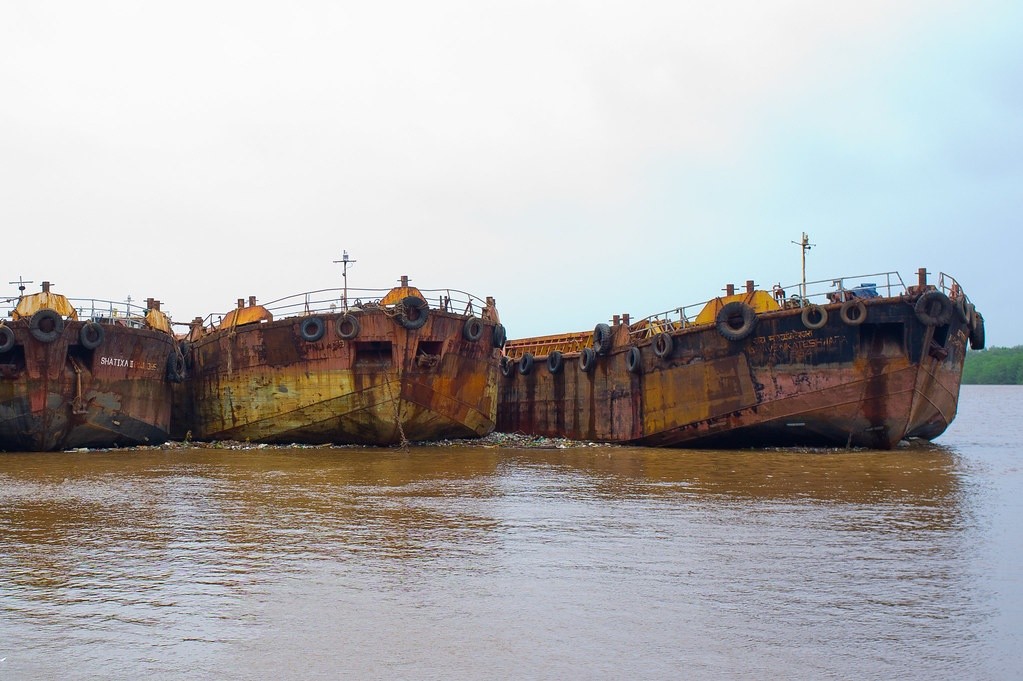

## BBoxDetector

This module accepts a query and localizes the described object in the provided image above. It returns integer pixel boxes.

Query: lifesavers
[519,353,533,375]
[463,316,483,342]
[801,305,828,330]
[397,296,429,330]
[301,317,324,342]
[626,347,640,373]
[651,332,673,358]
[716,301,758,342]
[29,308,64,344]
[580,347,593,372]
[840,300,867,326]
[594,323,611,355]
[168,352,186,383]
[546,351,563,374]
[79,322,105,350]
[0,324,15,353]
[915,290,985,350]
[493,325,506,348]
[501,356,514,378]
[335,314,359,340]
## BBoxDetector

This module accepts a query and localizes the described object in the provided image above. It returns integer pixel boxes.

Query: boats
[0,274,190,452]
[492,229,986,453]
[174,249,508,447]
